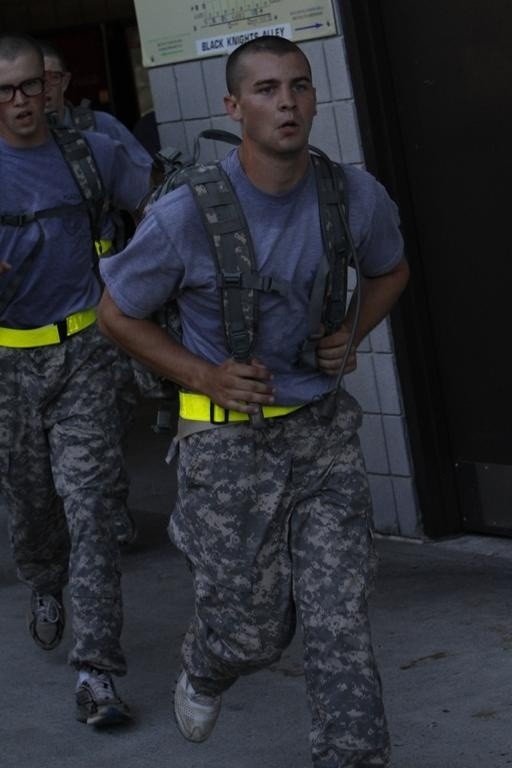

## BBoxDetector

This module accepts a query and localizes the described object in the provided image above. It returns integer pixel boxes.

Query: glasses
[3,76,43,104]
[46,72,68,86]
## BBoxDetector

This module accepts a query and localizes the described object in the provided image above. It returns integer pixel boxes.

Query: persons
[37,37,164,551]
[96,32,411,768]
[2,34,177,733]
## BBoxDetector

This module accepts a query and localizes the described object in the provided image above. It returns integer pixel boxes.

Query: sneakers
[74,670,135,727]
[25,580,66,650]
[111,505,136,544]
[173,663,223,744]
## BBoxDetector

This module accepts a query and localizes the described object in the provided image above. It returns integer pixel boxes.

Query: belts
[0,308,98,346]
[175,390,313,426]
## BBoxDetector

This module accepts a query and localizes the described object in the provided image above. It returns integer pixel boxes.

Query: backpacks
[130,125,354,369]
